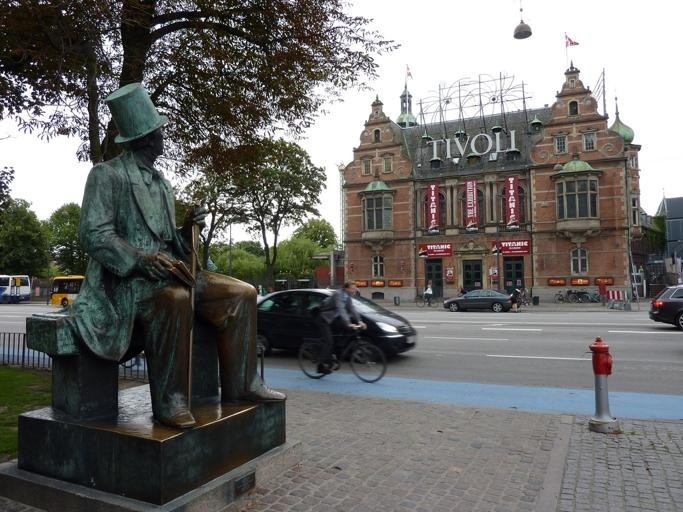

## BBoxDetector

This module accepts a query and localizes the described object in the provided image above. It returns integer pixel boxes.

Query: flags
[566,37,579,47]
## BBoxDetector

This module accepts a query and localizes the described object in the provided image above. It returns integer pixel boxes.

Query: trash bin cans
[394,297,400,306]
[533,296,539,305]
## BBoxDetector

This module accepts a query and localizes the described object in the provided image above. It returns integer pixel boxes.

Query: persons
[460,288,467,295]
[511,288,525,313]
[424,285,433,306]
[314,282,368,373]
[48,82,285,428]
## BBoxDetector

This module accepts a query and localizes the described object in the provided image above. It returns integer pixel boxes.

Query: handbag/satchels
[306,304,320,319]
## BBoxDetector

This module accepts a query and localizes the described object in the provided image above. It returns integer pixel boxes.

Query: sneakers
[317,363,332,374]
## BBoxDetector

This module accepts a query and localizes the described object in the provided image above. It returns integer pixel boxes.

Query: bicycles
[415,291,439,308]
[554,288,600,304]
[298,324,387,383]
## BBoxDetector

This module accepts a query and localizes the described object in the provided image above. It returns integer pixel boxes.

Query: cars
[648,285,683,330]
[443,287,522,312]
[257,288,417,363]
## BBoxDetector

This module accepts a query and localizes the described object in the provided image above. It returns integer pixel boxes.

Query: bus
[47,275,85,308]
[0,274,33,304]
[274,272,315,291]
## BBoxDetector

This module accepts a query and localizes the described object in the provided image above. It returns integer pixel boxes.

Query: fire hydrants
[589,336,620,434]
